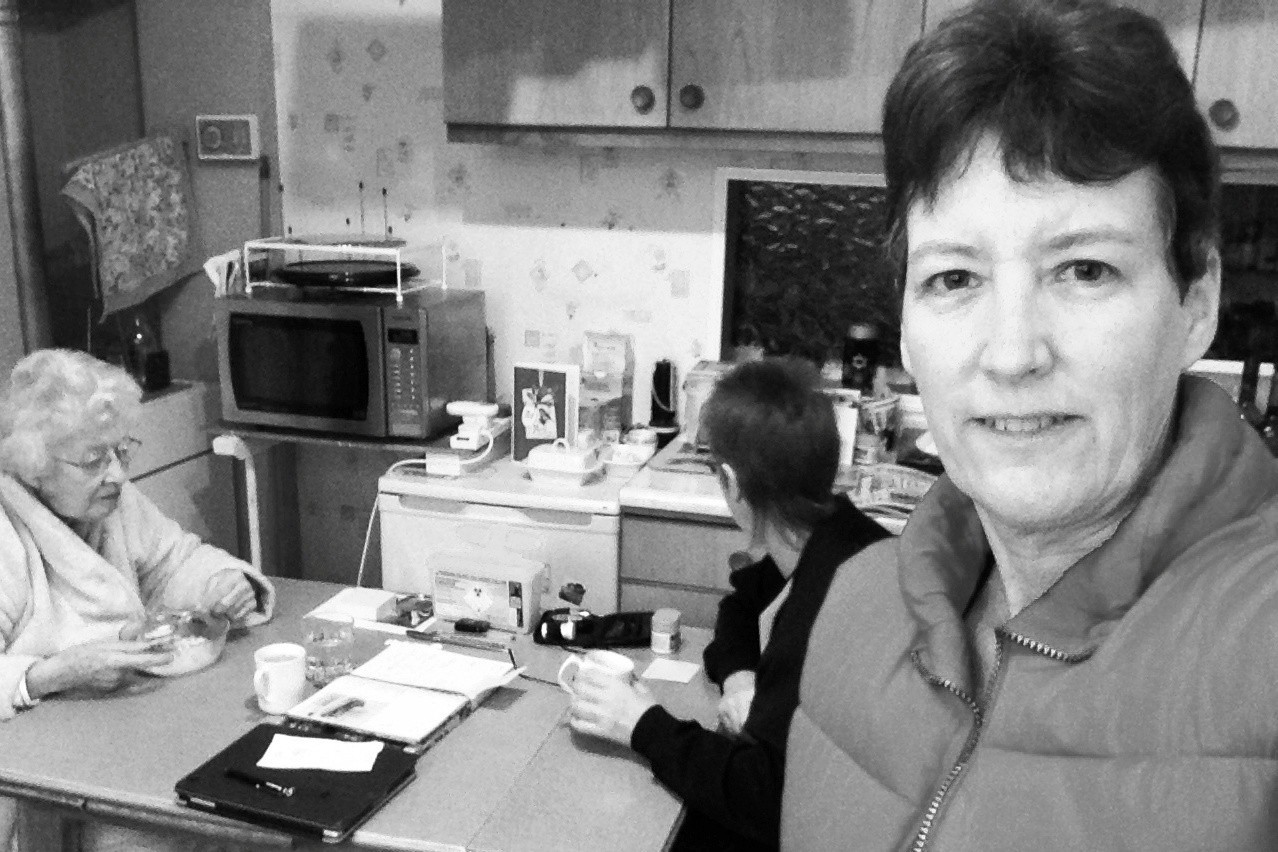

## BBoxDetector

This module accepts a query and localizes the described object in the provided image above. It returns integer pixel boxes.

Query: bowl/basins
[118,609,233,677]
[516,439,654,482]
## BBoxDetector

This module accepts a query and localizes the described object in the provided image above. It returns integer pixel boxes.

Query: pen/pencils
[224,767,295,797]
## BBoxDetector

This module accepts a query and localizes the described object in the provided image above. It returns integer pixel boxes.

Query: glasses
[46,437,142,476]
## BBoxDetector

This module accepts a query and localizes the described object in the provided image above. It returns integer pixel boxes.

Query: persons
[567,354,901,851]
[0,345,278,852]
[773,3,1277,849]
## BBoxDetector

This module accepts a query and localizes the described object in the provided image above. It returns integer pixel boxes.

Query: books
[281,639,514,755]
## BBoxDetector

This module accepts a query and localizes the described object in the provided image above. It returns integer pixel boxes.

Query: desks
[0,573,736,852]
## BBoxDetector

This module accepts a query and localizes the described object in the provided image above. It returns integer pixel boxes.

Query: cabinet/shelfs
[439,1,975,160]
[622,438,911,630]
[113,379,217,544]
[375,431,652,619]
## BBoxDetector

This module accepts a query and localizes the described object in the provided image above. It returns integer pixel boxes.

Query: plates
[272,260,418,287]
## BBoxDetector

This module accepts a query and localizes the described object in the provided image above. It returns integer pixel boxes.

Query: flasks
[842,322,880,395]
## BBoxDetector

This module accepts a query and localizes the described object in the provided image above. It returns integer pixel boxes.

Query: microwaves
[212,287,489,440]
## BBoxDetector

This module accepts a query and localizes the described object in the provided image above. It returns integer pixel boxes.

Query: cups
[254,642,304,719]
[302,614,355,685]
[558,650,632,695]
[624,424,678,451]
